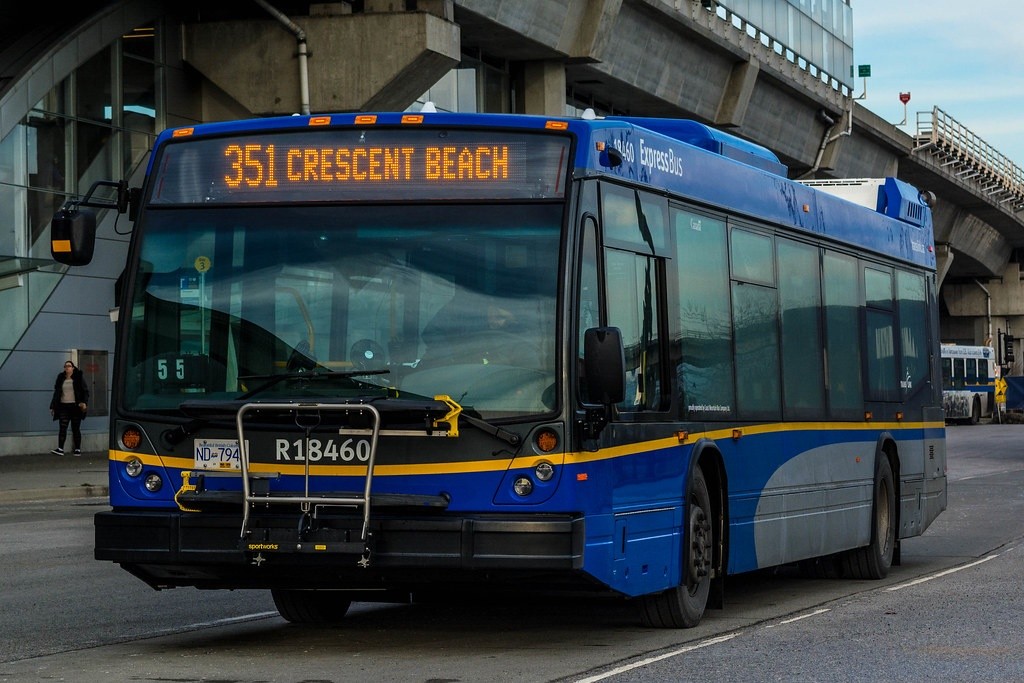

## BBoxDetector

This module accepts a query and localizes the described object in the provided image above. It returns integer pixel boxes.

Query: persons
[419,282,526,352]
[49,360,91,457]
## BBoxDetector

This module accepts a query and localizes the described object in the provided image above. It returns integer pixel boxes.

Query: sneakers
[73,450,82,456]
[50,447,64,456]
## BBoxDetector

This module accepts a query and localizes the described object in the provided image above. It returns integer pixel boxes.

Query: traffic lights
[1002,368,1011,376]
[1006,335,1014,362]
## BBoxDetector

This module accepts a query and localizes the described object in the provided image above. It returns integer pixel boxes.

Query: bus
[940,345,995,426]
[49,102,949,629]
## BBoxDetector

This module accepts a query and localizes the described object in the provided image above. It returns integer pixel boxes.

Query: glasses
[64,365,72,368]
